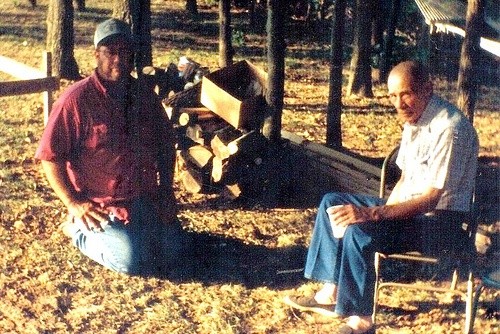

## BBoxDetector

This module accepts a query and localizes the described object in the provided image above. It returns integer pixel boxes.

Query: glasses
[98,48,129,58]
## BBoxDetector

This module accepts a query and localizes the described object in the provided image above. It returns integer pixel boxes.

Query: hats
[94,18,132,50]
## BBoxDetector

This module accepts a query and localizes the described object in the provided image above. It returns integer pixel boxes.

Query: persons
[283,59,480,334]
[33,18,191,275]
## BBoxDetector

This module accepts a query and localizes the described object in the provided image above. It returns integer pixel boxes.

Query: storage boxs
[201,59,271,129]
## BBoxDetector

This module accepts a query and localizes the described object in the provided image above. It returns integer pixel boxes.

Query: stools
[469,266,500,334]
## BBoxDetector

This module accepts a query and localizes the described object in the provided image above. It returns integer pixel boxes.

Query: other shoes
[336,323,376,334]
[285,295,339,318]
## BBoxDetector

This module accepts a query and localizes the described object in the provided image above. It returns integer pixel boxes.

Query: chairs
[371,140,479,334]
